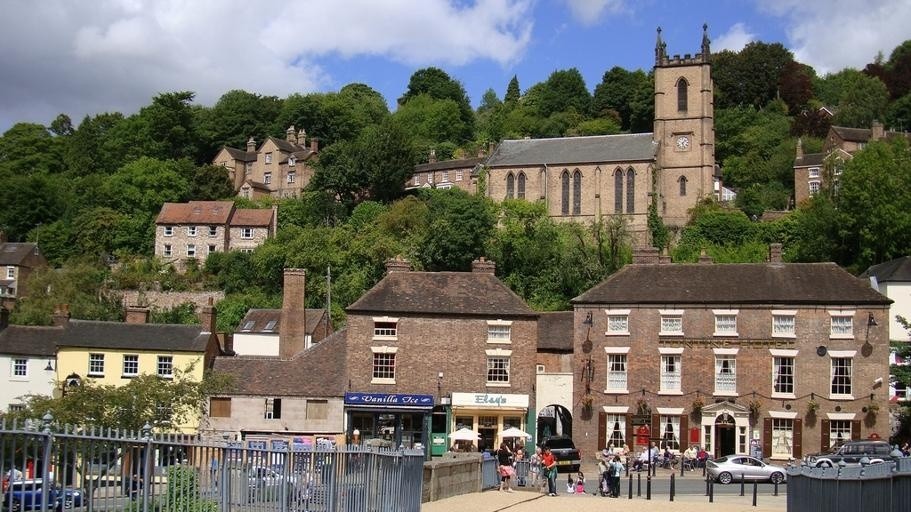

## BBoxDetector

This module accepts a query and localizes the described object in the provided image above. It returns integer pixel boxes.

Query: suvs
[4,477,84,512]
[801,439,894,469]
[214,465,313,500]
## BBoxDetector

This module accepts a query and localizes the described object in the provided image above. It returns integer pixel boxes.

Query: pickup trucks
[535,435,583,473]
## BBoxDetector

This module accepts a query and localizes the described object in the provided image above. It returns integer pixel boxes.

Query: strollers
[599,472,614,498]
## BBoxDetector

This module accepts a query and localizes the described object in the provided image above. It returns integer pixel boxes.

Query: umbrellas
[497,425,531,449]
[448,428,484,441]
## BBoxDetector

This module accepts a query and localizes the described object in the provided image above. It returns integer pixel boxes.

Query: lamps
[436,371,443,390]
[860,311,879,341]
[582,311,593,330]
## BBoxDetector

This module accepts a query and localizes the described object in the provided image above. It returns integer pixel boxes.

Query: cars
[705,453,786,484]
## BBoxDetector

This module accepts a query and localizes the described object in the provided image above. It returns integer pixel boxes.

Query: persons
[891,442,911,456]
[5,464,24,481]
[353,425,361,444]
[498,441,709,499]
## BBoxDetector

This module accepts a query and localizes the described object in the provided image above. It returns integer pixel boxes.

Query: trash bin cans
[516,461,529,487]
